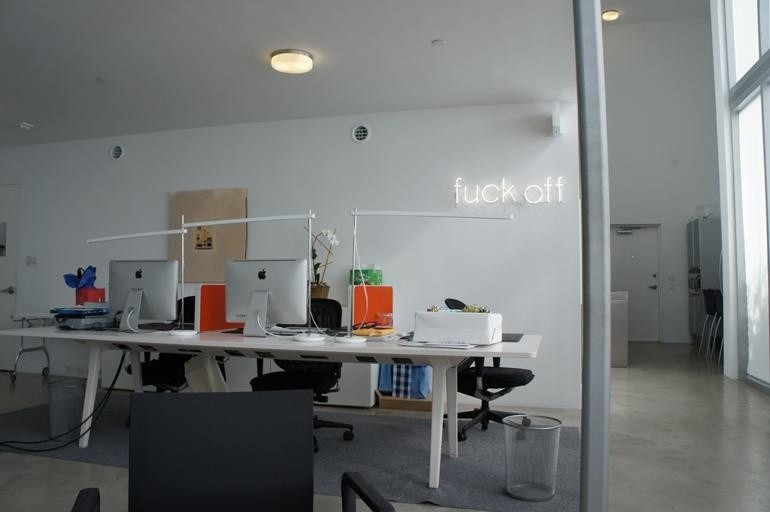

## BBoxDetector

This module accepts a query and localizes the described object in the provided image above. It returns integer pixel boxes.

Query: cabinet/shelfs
[685,214,723,338]
[271,306,379,409]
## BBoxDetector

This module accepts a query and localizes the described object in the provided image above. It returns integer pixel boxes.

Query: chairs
[64,386,395,512]
[697,288,717,358]
[248,295,357,452]
[711,287,724,365]
[443,297,538,442]
[122,294,223,432]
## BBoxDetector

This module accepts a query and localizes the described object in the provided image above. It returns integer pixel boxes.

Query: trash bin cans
[502,415,562,502]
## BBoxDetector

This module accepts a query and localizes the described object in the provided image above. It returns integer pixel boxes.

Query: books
[47,302,109,330]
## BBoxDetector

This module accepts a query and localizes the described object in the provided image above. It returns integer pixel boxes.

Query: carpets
[0,391,583,512]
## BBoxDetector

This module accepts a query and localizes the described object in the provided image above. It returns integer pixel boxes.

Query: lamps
[267,46,314,77]
[601,7,622,23]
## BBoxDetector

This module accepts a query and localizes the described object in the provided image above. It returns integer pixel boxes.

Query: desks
[0,326,544,492]
[10,310,90,390]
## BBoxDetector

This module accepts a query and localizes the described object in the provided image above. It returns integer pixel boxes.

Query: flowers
[310,227,339,285]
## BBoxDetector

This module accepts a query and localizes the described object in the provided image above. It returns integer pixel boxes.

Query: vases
[311,283,330,299]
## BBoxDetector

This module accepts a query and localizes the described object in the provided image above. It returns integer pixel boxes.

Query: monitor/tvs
[108,260,178,333]
[226,258,307,337]
